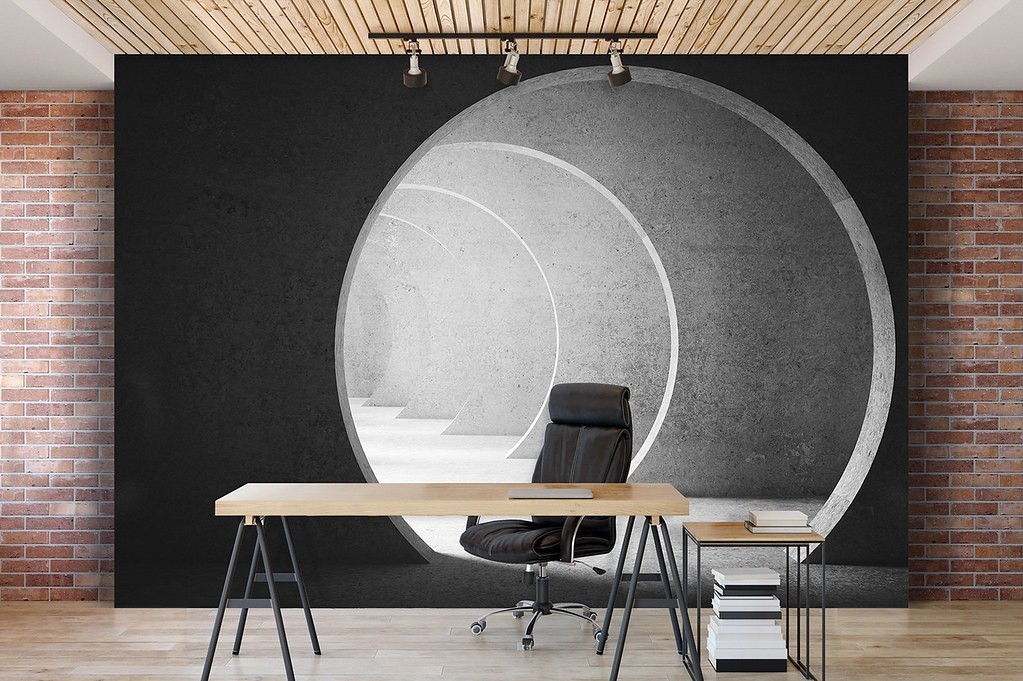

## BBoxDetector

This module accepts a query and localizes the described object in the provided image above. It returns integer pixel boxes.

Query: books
[744,511,812,534]
[707,568,788,672]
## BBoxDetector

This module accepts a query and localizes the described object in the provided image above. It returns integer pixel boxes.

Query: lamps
[497,36,521,85]
[607,40,632,86]
[403,37,428,88]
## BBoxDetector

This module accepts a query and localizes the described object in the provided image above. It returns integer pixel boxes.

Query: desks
[197,483,703,681]
[680,520,826,680]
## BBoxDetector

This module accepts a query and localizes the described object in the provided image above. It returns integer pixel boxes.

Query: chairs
[460,384,633,655]
